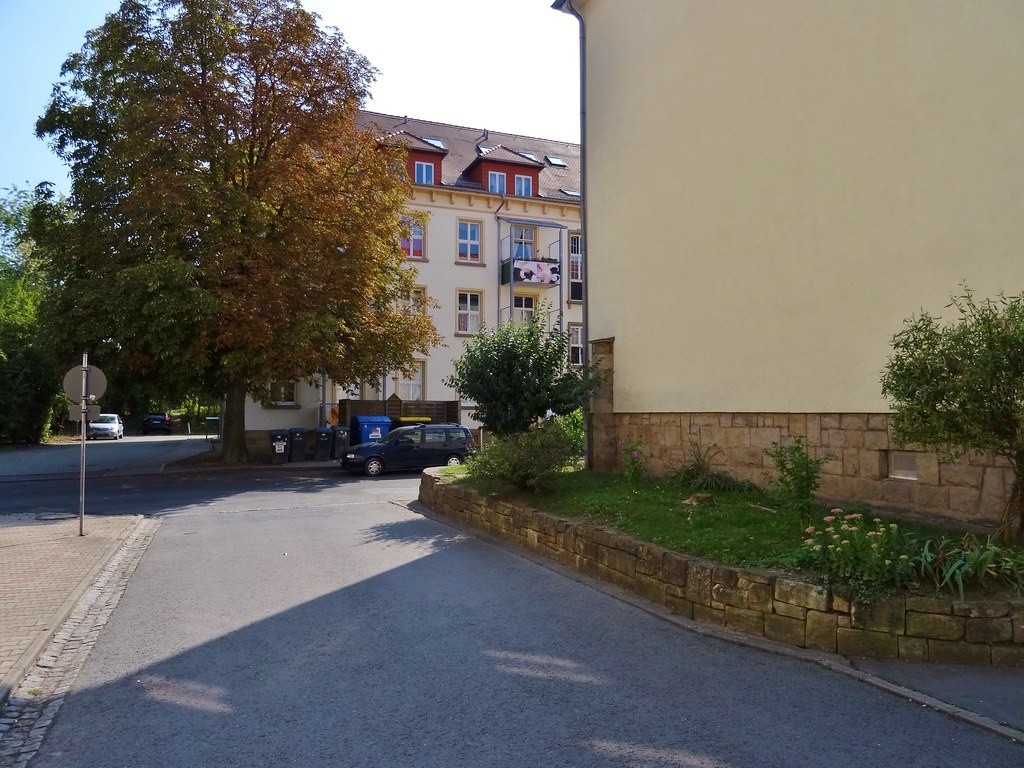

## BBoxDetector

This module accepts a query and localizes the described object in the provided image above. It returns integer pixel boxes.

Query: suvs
[340,421,478,476]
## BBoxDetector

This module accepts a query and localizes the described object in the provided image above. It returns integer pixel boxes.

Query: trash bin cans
[399,417,432,427]
[331,426,350,460]
[289,427,309,463]
[312,428,332,462]
[269,429,289,465]
[355,415,392,445]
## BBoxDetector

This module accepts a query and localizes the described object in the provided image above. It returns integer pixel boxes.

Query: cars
[142,411,173,435]
[87,414,124,440]
